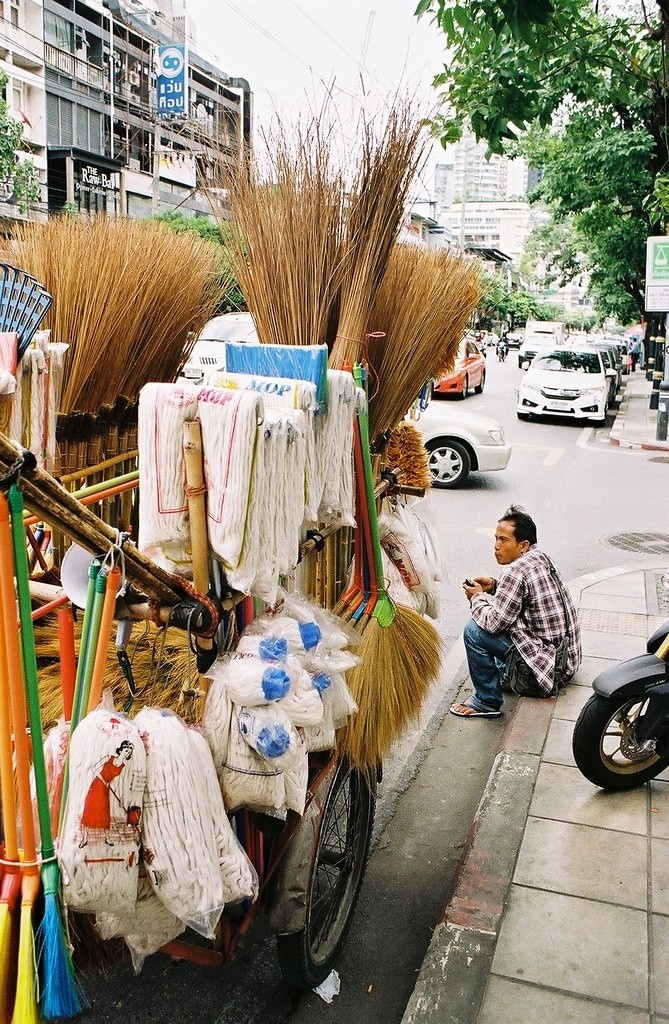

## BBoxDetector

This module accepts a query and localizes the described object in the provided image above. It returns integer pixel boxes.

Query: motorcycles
[571,615,669,792]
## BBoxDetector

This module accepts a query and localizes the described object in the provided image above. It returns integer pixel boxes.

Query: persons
[474,331,487,359]
[627,337,639,372]
[451,504,581,719]
[495,331,509,358]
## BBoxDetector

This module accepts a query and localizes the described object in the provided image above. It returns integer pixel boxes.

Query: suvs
[518,333,559,369]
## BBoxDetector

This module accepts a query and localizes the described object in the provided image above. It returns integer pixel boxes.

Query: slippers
[449,700,502,716]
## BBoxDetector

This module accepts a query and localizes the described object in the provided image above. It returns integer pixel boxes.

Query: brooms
[0,36,522,1024]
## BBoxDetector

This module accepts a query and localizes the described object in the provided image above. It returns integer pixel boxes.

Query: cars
[487,333,498,345]
[175,312,264,385]
[516,331,632,427]
[398,394,512,490]
[505,328,525,345]
[419,339,486,400]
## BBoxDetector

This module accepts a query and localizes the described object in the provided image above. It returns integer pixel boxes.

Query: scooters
[497,344,506,362]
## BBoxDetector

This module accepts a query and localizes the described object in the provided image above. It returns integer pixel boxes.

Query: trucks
[526,321,565,346]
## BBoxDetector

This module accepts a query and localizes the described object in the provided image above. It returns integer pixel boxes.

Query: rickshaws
[68,697,383,991]
